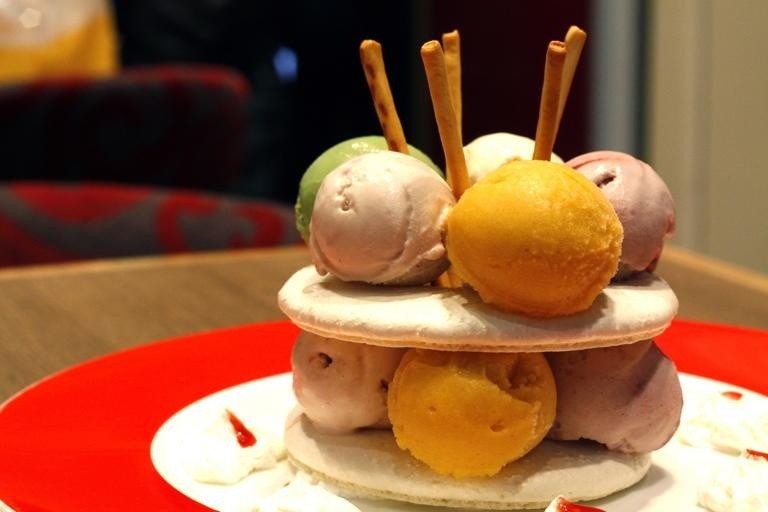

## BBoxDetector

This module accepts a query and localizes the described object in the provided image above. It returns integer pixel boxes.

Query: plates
[148,369,768,511]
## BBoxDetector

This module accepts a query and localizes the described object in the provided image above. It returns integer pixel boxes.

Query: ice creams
[276,25,683,511]
